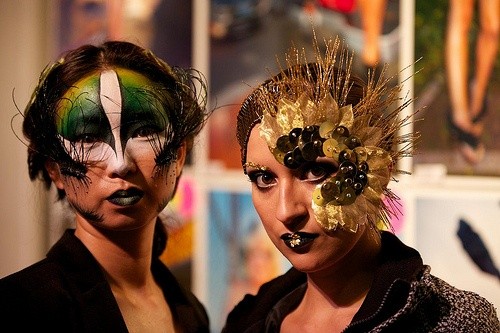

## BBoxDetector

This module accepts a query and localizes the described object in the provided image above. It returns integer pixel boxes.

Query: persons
[0,40,211,333]
[315,0,388,82]
[446,0,499,147]
[219,62,500,333]
